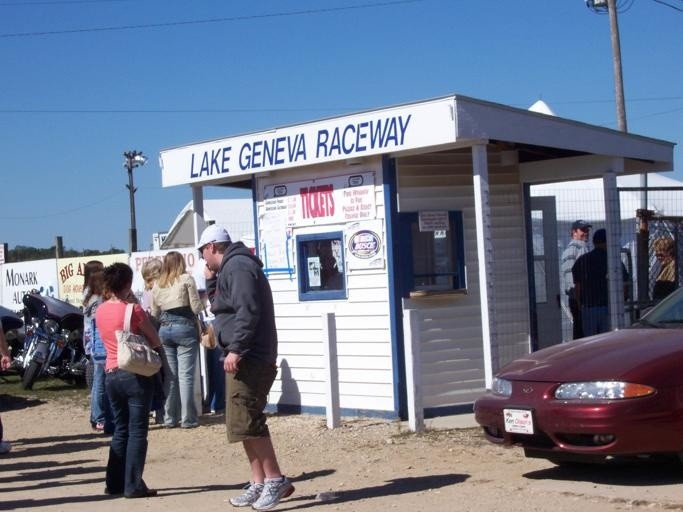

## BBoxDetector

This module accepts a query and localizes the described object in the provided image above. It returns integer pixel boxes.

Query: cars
[473,286,683,467]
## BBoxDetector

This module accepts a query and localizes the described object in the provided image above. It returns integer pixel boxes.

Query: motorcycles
[22,288,94,390]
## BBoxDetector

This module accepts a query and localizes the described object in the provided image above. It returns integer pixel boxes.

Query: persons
[199,223,294,510]
[0,305,12,454]
[152,252,204,430]
[571,229,626,338]
[95,263,159,498]
[84,270,112,433]
[140,259,163,418]
[652,239,676,301]
[82,260,102,390]
[559,219,592,339]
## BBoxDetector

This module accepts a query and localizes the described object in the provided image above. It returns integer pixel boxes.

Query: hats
[198,225,230,249]
[573,219,591,230]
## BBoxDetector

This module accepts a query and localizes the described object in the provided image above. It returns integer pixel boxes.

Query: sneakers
[148,416,199,428]
[229,483,264,506]
[92,423,104,429]
[143,489,156,496]
[203,405,211,413]
[252,475,294,510]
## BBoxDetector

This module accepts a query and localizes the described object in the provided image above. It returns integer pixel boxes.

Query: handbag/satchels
[199,320,216,348]
[91,318,106,360]
[116,330,163,377]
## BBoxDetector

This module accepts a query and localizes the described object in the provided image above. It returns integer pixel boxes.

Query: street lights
[123,150,147,251]
